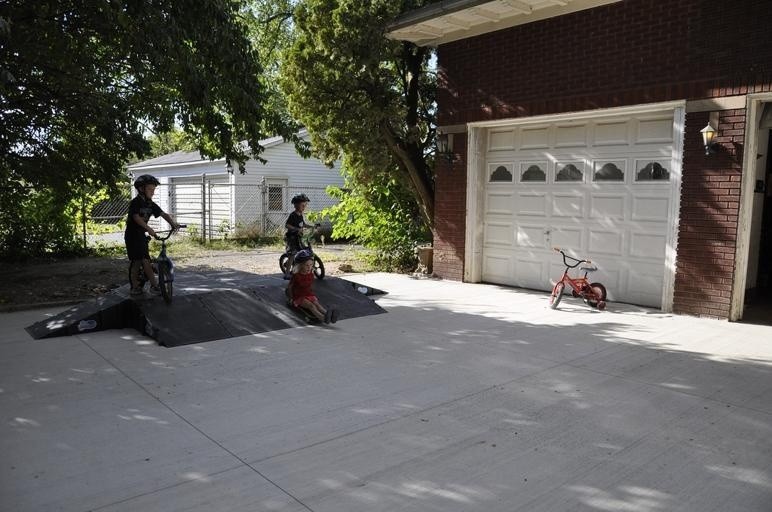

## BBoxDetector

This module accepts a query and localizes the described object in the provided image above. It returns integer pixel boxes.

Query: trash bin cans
[417,244,434,274]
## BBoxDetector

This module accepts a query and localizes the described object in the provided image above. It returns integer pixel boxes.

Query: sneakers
[331,309,337,323]
[132,287,142,295]
[150,286,161,294]
[324,309,333,324]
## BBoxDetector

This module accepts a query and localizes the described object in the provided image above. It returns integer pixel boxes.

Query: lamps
[699,110,723,159]
[433,129,456,162]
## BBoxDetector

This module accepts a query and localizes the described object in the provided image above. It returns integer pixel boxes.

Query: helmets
[134,175,160,189]
[295,249,313,261]
[291,193,310,204]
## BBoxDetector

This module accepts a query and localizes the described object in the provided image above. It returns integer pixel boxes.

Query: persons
[285,249,339,324]
[125,174,179,296]
[283,193,318,279]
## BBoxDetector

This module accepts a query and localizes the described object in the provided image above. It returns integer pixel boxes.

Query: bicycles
[127,224,187,304]
[548,247,607,311]
[279,222,325,280]
